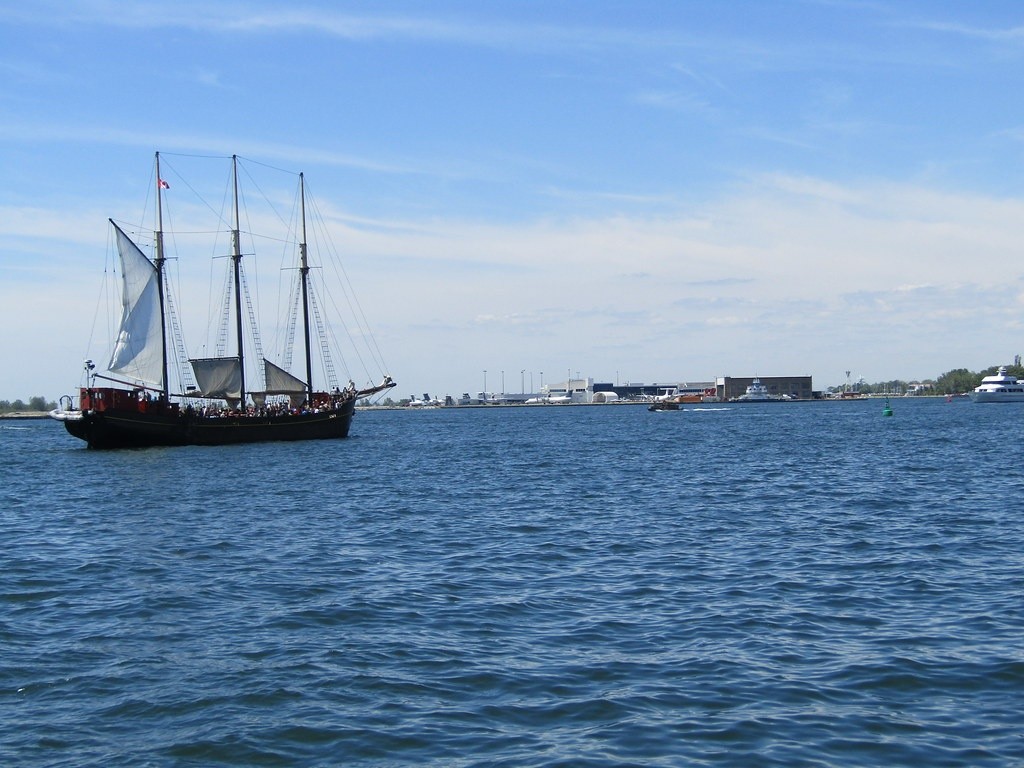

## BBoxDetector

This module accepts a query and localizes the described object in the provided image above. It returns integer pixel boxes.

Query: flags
[158,178,170,190]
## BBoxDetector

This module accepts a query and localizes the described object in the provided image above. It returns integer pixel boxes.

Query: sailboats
[49,151,397,451]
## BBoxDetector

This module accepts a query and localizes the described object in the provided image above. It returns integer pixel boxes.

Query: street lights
[501,370,504,396]
[483,370,487,392]
[520,369,526,394]
[540,371,543,387]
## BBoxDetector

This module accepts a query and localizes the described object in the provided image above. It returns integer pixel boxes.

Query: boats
[728,372,792,402]
[967,366,1024,402]
[647,399,683,411]
[49,408,97,422]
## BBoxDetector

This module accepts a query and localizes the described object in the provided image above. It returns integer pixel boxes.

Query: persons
[142,379,358,418]
